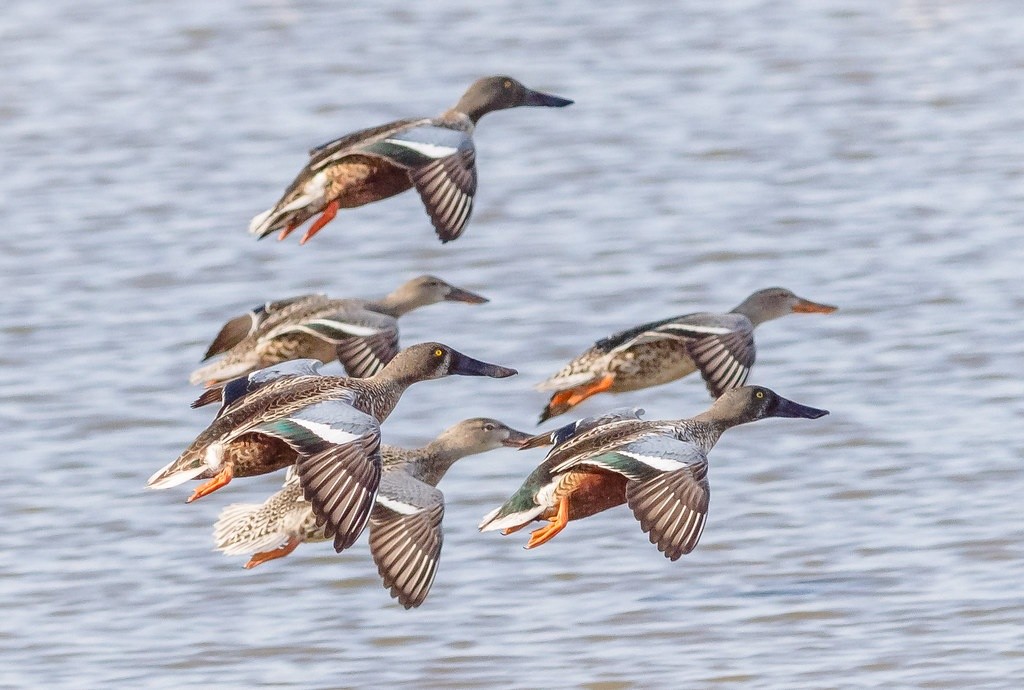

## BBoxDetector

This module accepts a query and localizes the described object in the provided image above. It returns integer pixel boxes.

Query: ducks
[246,74,577,244]
[190,274,492,409]
[142,341,520,555]
[477,385,830,562]
[211,416,537,611]
[538,288,839,425]
